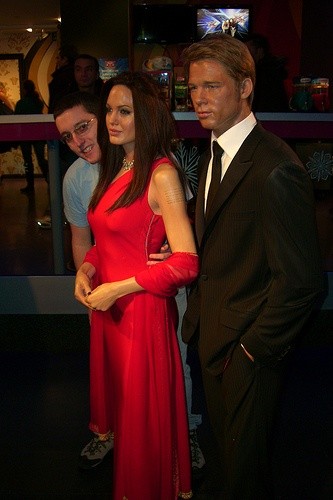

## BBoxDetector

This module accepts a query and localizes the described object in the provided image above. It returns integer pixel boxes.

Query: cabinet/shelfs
[128,0,195,73]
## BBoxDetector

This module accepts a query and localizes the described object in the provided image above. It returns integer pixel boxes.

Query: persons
[70,55,105,112]
[250,35,290,111]
[13,78,52,194]
[74,73,199,500]
[54,92,206,471]
[48,45,88,114]
[180,34,329,500]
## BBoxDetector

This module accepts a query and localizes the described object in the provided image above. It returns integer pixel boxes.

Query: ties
[206,140,224,214]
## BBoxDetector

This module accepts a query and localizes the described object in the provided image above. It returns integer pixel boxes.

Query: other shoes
[81,435,114,467]
[191,442,206,471]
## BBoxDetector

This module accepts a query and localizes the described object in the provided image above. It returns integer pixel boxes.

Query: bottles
[288,77,311,112]
[311,78,330,112]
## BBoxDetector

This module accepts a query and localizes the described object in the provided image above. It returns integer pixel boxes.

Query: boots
[39,161,50,185]
[19,166,35,193]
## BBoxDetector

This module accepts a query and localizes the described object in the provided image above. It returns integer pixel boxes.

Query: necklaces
[122,155,136,172]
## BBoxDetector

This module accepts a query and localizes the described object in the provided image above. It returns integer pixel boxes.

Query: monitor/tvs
[194,5,252,41]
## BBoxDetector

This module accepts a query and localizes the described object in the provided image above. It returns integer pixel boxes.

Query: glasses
[61,115,97,144]
